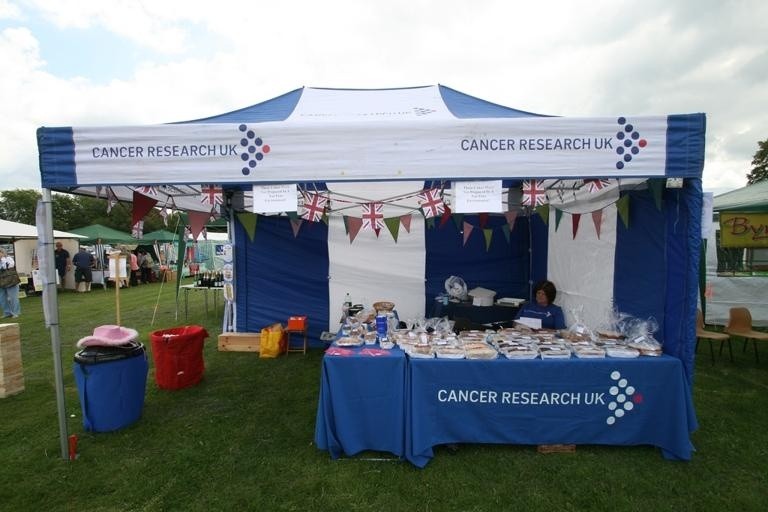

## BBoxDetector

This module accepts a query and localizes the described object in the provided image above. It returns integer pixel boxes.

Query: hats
[75,323,141,349]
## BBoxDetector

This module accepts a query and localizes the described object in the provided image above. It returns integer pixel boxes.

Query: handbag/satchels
[258,321,288,359]
[0,266,21,290]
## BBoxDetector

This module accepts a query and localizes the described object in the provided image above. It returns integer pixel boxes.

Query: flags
[200,183,223,205]
[134,185,160,197]
[207,207,220,222]
[105,185,118,214]
[300,190,328,223]
[359,202,385,232]
[584,176,613,195]
[132,218,144,241]
[158,205,170,228]
[416,187,446,220]
[183,225,191,244]
[522,178,546,207]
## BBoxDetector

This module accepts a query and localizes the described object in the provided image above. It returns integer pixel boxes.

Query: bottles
[194,270,224,287]
[345,293,352,313]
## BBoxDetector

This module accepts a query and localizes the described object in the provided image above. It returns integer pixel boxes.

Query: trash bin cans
[148,325,209,391]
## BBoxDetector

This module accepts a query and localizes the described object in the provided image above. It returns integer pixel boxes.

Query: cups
[376,315,387,343]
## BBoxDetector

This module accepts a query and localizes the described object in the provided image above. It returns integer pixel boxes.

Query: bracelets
[67,265,71,268]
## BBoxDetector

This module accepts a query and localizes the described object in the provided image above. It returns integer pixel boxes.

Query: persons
[70,244,98,293]
[55,241,72,292]
[100,248,154,288]
[0,247,21,319]
[512,280,567,330]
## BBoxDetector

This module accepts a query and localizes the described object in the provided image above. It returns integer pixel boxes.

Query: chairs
[698,308,768,366]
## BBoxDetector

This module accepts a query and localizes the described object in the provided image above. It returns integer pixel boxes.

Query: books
[512,316,544,331]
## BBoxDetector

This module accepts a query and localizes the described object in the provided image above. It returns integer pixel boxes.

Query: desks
[316,305,698,469]
[705,272,768,327]
[178,280,233,324]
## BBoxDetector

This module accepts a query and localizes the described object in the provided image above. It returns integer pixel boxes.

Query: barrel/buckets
[72,339,150,435]
